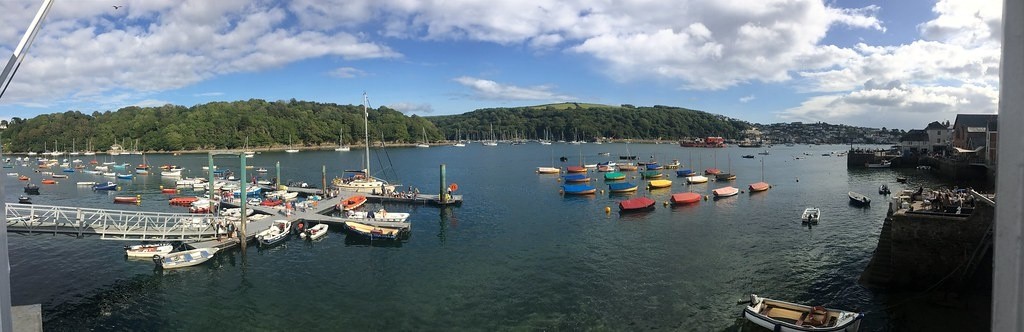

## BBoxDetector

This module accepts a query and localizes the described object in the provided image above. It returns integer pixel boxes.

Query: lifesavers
[311,229,316,235]
[153,255,160,263]
[450,183,458,191]
[809,306,828,326]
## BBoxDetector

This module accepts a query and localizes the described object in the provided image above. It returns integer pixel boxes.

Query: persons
[216,221,239,242]
[911,184,924,202]
[312,200,318,211]
[921,186,976,214]
[389,185,420,200]
[447,184,453,198]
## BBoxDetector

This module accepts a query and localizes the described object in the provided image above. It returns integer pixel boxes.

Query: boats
[878,183,892,195]
[711,186,739,197]
[2,143,418,245]
[847,190,872,206]
[802,205,820,224]
[535,154,738,194]
[916,165,932,170]
[741,150,848,160]
[678,136,729,150]
[738,137,772,148]
[418,133,613,150]
[154,247,219,270]
[747,182,770,193]
[669,189,702,206]
[617,194,657,214]
[121,242,172,259]
[735,292,863,332]
[896,176,907,183]
[331,91,403,193]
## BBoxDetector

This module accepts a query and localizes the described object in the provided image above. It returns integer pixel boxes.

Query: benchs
[201,251,209,258]
[827,316,836,327]
[184,254,193,261]
[762,305,774,315]
[166,258,172,262]
[795,312,809,326]
